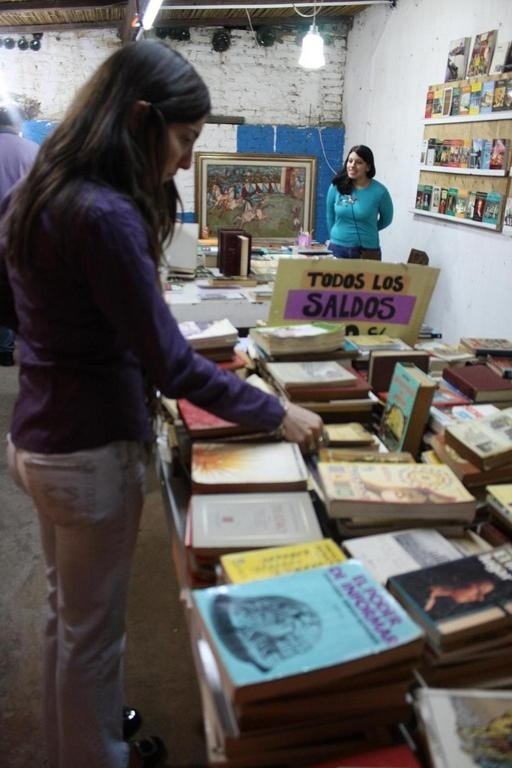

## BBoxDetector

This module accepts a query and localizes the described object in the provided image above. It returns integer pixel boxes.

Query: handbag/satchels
[360,248,381,259]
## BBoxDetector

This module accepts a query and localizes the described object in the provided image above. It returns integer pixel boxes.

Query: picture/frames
[194,151,317,248]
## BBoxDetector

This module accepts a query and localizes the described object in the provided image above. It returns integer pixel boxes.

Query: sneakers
[0,351,14,366]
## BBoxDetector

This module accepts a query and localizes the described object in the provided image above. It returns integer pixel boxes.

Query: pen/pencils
[300,224,314,236]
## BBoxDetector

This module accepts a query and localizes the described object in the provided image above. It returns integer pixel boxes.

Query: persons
[0,103,42,368]
[325,146,393,261]
[0,39,332,768]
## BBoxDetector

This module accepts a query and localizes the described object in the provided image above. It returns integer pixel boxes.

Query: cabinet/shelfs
[409,72,511,232]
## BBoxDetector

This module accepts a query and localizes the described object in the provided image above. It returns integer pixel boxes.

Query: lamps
[295,0,325,73]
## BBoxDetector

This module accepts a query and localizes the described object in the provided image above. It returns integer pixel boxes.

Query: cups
[298,231,312,247]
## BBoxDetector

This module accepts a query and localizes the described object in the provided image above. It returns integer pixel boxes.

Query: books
[158,223,512,767]
[421,138,512,170]
[416,184,504,224]
[425,30,512,118]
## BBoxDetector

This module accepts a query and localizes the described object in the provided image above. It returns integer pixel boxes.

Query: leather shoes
[121,708,165,767]
